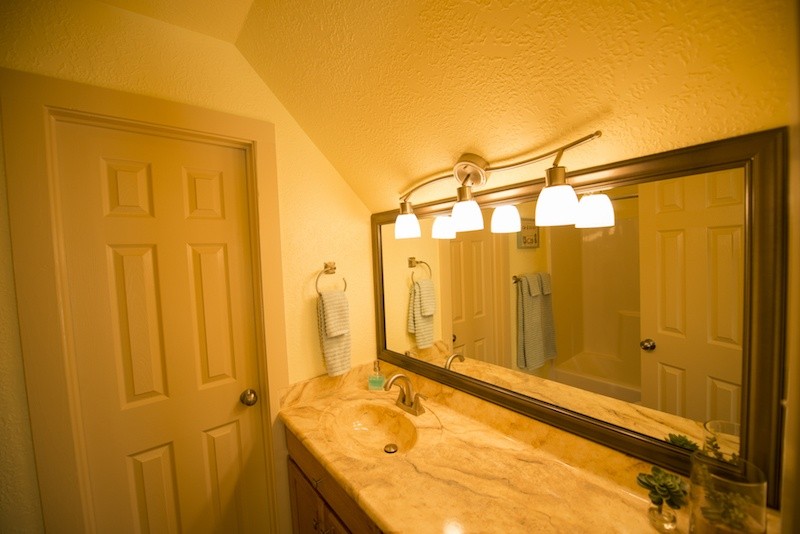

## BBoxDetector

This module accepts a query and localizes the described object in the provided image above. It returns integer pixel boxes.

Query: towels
[316,289,352,377]
[517,271,556,373]
[407,278,438,349]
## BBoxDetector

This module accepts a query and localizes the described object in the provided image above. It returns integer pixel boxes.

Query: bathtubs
[558,352,642,389]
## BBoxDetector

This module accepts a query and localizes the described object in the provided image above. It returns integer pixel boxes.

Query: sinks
[326,401,419,458]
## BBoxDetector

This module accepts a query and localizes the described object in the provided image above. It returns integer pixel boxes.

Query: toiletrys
[368,360,385,390]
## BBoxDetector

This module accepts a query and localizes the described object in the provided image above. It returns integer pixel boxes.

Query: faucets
[444,352,466,371]
[383,372,429,417]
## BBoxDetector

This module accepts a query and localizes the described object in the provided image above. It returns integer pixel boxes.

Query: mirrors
[371,128,789,512]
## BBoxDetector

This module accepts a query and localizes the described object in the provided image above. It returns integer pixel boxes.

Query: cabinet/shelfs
[284,427,382,534]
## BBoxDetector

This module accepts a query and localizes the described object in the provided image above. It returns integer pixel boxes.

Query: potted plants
[686,438,767,534]
[636,469,687,534]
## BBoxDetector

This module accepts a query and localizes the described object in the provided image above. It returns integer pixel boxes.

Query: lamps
[431,193,615,240]
[394,132,614,240]
